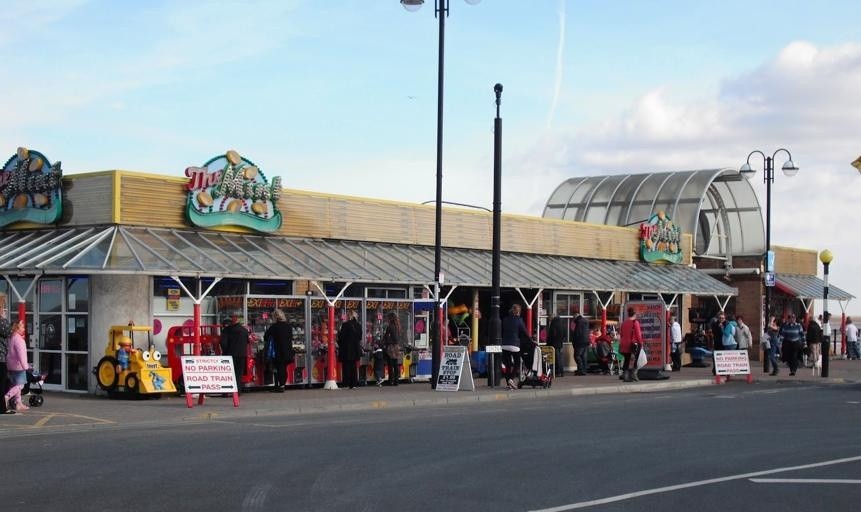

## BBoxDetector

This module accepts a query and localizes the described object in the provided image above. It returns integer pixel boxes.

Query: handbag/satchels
[267,336,276,358]
[632,341,640,351]
[338,342,358,361]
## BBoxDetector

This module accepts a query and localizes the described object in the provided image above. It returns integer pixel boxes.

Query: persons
[2,320,31,412]
[219,318,249,398]
[336,309,362,390]
[712,311,861,377]
[668,315,683,372]
[618,306,644,383]
[546,312,565,377]
[500,303,532,391]
[0,292,17,414]
[569,311,589,376]
[370,338,386,386]
[379,313,404,387]
[262,308,293,394]
[116,336,132,374]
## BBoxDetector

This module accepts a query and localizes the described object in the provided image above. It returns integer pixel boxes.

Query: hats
[275,311,285,319]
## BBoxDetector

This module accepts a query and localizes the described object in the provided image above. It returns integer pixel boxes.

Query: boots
[624,370,632,382]
[770,363,778,376]
[631,369,639,381]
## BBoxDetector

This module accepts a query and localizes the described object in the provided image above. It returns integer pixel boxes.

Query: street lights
[818,248,834,379]
[399,0,482,390]
[738,148,800,374]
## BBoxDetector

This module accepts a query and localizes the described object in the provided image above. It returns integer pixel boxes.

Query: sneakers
[15,403,30,411]
[376,379,398,386]
[575,369,587,375]
[507,379,518,390]
[270,386,284,393]
[3,395,9,409]
[557,371,566,377]
[789,371,796,376]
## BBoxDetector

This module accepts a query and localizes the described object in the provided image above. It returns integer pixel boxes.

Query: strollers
[8,363,48,411]
[516,340,552,392]
[595,333,622,377]
